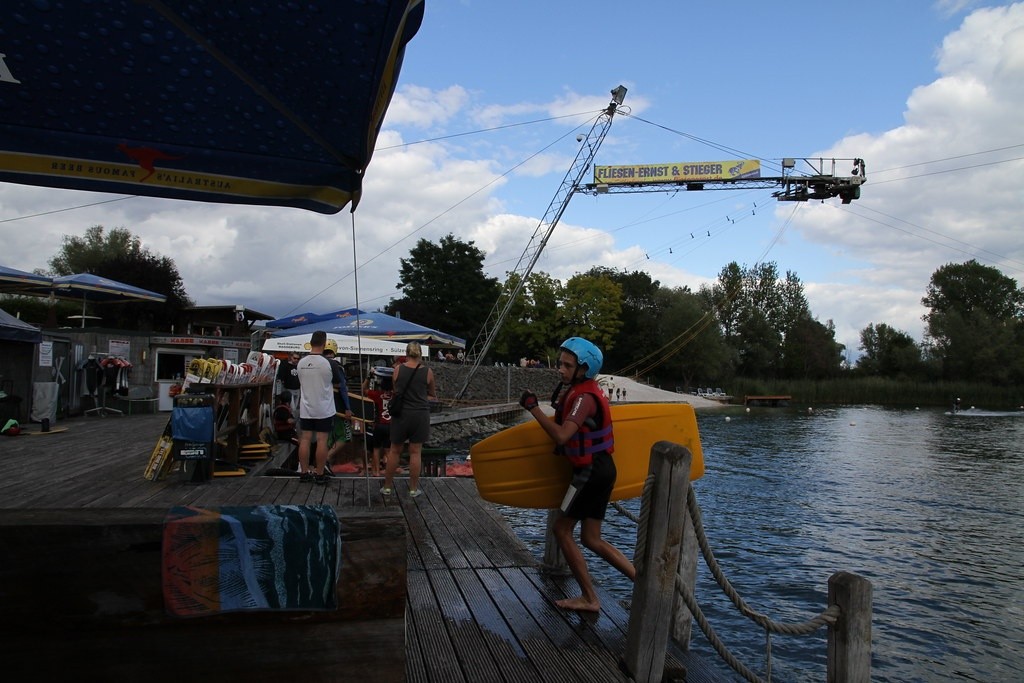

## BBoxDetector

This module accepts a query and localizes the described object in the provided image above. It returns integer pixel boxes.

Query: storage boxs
[129,398,160,414]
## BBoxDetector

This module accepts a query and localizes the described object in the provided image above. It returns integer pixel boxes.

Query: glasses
[292,356,300,360]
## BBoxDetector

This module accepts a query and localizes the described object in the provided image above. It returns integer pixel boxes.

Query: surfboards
[469,403,705,509]
[332,388,376,423]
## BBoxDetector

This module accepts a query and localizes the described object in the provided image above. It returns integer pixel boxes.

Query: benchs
[0,508,407,683]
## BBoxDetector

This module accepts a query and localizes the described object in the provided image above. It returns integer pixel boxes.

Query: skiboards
[143,351,281,483]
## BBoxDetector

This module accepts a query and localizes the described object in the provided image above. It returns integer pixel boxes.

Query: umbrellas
[265,307,365,329]
[49,273,166,329]
[0,0,425,216]
[270,311,466,374]
[0,267,53,298]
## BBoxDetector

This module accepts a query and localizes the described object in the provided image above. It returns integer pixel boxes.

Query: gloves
[367,372,375,380]
[519,391,538,410]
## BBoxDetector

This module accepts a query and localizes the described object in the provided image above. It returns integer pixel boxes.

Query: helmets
[323,340,337,356]
[561,336,603,379]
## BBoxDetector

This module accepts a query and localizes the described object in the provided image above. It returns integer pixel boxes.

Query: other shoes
[410,488,422,497]
[300,471,313,481]
[379,487,391,495]
[324,462,333,475]
[315,475,330,483]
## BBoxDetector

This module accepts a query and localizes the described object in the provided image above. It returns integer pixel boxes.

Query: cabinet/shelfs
[79,352,124,417]
[189,383,273,480]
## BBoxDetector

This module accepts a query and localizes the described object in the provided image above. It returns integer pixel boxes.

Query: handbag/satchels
[389,396,403,417]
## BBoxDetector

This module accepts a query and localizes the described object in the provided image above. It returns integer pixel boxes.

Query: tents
[0,308,43,422]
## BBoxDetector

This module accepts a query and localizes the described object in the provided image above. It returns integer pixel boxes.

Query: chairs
[676,386,726,396]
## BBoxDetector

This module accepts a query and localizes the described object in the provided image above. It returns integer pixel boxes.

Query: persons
[380,340,436,498]
[437,349,446,363]
[321,348,354,476]
[275,353,302,416]
[457,350,464,364]
[520,357,540,368]
[362,371,396,477]
[297,332,342,486]
[520,337,635,611]
[214,326,222,337]
[446,350,454,363]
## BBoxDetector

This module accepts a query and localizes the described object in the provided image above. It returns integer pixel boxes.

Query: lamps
[611,85,627,104]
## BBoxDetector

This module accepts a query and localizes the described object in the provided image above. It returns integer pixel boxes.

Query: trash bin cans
[173,393,216,460]
